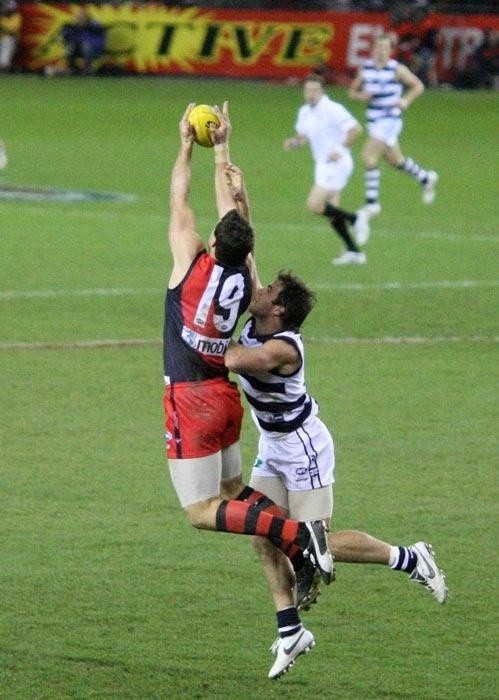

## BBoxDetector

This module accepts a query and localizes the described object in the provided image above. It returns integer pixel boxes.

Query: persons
[0,2,24,73]
[282,74,370,267]
[160,99,336,611]
[222,158,448,680]
[471,25,499,89]
[347,33,438,218]
[61,11,105,77]
[393,7,442,89]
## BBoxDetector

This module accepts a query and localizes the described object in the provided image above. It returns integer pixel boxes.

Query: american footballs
[189,105,221,147]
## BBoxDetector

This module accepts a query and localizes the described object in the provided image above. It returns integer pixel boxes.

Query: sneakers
[352,211,370,246]
[423,171,438,204]
[268,621,316,681]
[301,519,336,585]
[362,202,381,217]
[333,250,367,265]
[293,556,322,611]
[408,542,449,605]
